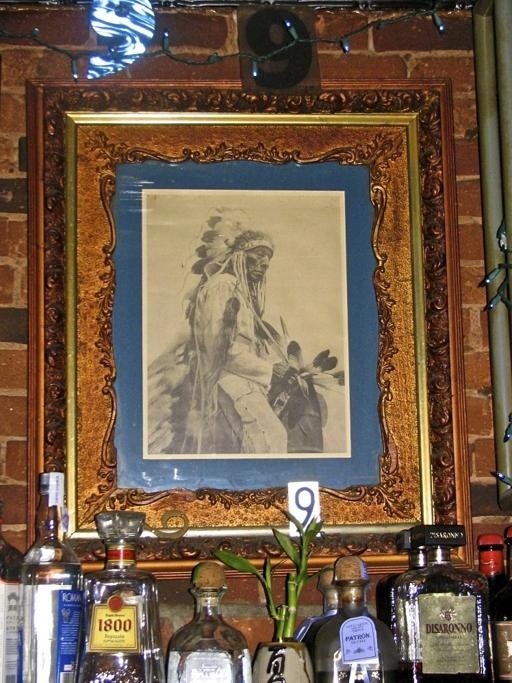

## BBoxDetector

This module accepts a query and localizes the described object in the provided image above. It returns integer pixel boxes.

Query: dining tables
[211,509,332,681]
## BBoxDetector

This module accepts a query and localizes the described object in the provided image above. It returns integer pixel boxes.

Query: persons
[184,231,308,455]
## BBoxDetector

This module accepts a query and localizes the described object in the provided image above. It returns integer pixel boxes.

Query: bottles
[2,538,24,680]
[162,561,252,682]
[296,525,512,683]
[18,473,85,681]
[77,510,169,682]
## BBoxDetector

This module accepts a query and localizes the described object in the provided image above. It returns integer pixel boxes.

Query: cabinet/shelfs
[296,525,512,683]
